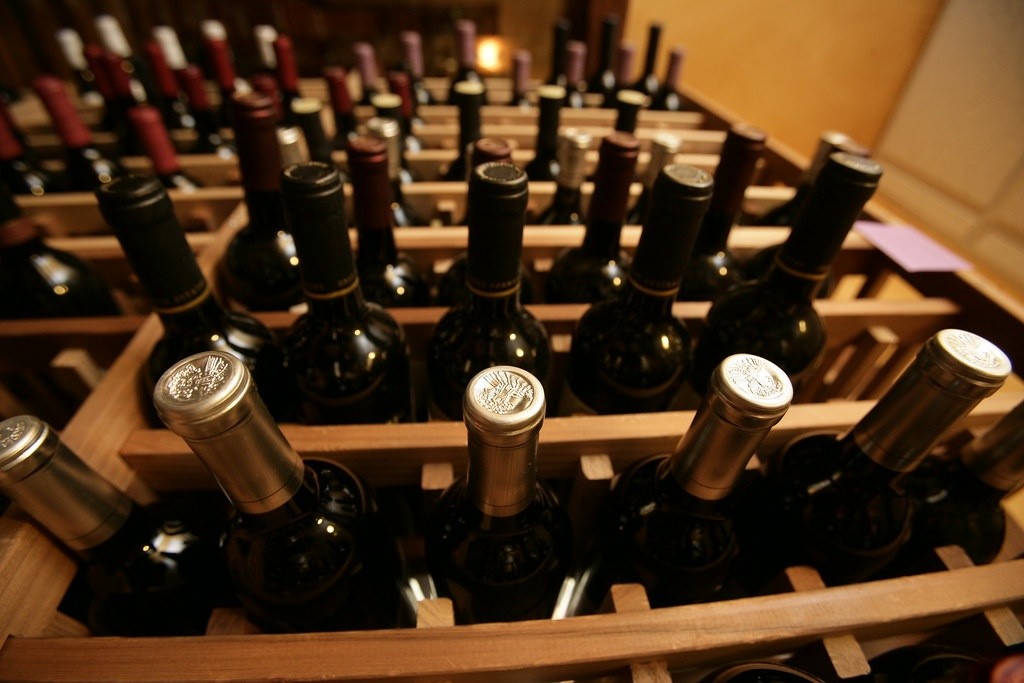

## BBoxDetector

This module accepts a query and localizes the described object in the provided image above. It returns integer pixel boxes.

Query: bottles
[0,14,1024,683]
